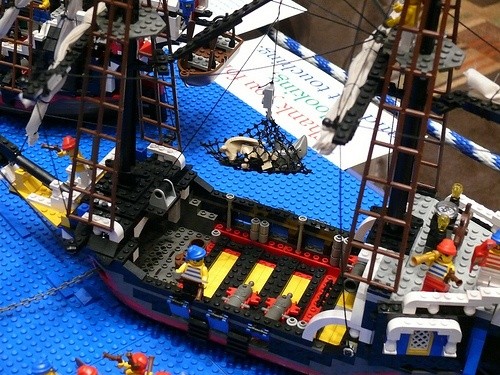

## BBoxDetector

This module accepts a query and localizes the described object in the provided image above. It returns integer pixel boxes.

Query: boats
[1,0,477,375]
